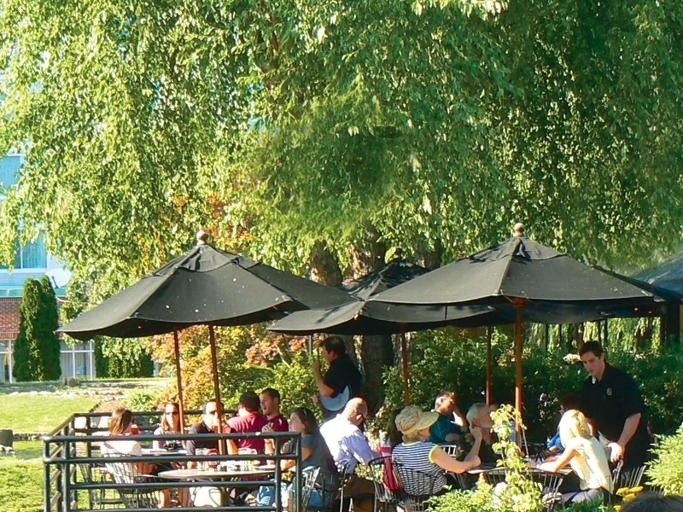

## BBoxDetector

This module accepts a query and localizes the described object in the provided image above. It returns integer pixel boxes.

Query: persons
[103,408,179,500]
[318,397,381,473]
[391,390,516,510]
[546,395,613,512]
[579,342,649,486]
[187,401,238,470]
[312,336,361,421]
[153,403,181,451]
[230,389,338,509]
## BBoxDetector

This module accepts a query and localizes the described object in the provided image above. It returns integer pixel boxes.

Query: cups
[195,447,206,470]
[130,427,138,434]
[163,431,174,449]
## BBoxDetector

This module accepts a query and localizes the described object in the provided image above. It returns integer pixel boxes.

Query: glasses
[295,406,308,417]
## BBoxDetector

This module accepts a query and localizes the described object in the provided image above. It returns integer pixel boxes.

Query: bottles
[206,447,257,472]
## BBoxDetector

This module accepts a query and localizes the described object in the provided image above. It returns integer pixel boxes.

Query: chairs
[93,394,683,511]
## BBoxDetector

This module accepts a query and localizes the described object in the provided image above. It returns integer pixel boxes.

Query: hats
[396,404,439,435]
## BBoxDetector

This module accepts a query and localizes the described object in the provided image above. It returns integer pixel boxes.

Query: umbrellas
[367,223,666,443]
[266,247,497,409]
[54,231,363,502]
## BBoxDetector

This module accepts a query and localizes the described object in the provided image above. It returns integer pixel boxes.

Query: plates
[255,464,274,469]
